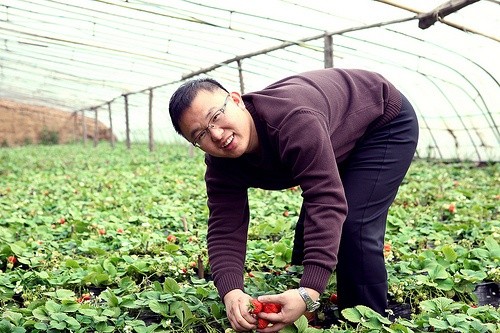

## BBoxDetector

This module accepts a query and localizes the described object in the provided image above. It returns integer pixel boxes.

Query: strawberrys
[329,204,477,309]
[8,211,293,303]
[248,299,282,329]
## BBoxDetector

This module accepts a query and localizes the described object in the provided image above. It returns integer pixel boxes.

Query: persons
[168,68,419,333]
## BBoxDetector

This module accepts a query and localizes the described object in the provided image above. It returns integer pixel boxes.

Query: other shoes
[316,308,390,330]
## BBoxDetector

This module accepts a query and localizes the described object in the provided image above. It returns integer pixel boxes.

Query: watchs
[297,286,320,312]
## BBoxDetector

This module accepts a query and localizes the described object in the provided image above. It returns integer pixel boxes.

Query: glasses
[191,95,230,148]
